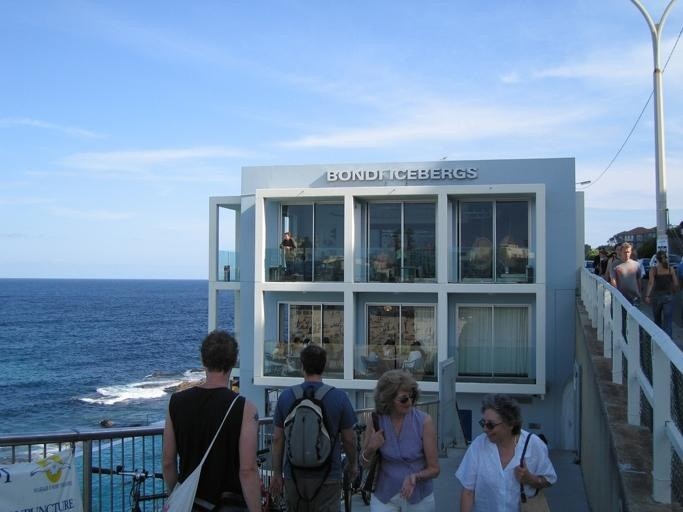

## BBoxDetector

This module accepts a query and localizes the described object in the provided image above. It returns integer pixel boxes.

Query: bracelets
[359,455,366,465]
[361,450,369,464]
[416,470,422,480]
[413,473,420,482]
[533,476,541,490]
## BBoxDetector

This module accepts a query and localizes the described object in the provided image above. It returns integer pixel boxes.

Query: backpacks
[283,385,335,468]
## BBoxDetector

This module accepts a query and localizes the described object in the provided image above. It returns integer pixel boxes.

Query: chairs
[360,353,431,380]
[264,351,302,377]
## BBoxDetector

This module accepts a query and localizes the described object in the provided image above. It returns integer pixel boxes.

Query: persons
[452,392,559,512]
[582,240,682,347]
[276,230,434,379]
[269,346,360,511]
[160,327,263,511]
[355,368,440,512]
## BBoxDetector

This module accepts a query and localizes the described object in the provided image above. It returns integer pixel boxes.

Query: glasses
[479,420,502,428]
[400,393,414,403]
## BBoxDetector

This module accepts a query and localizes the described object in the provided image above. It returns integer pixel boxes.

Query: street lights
[575,181,592,186]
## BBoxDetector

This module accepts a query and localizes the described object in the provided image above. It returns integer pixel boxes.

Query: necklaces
[199,382,226,387]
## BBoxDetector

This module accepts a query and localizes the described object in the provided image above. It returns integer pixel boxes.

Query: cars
[584,260,594,273]
[639,258,651,275]
[649,254,682,273]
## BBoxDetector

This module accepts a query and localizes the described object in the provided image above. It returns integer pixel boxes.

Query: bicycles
[89,421,372,510]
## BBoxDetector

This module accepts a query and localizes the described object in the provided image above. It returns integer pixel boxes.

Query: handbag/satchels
[163,465,202,512]
[520,488,550,512]
[360,449,380,505]
